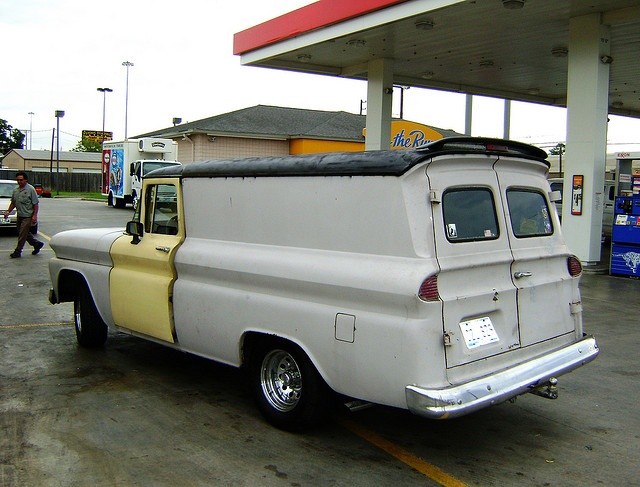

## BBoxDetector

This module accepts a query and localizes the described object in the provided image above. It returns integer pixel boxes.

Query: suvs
[48,136,599,434]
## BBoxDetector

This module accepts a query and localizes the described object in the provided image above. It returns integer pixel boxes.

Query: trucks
[101,138,178,208]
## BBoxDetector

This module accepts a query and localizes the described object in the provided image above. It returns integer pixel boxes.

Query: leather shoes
[32,242,44,255]
[11,252,21,258]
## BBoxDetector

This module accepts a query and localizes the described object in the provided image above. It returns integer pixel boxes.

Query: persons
[4,171,44,258]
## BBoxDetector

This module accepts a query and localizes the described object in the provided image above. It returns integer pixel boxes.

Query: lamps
[297,54,312,61]
[526,87,541,95]
[414,20,434,31]
[551,49,567,58]
[420,71,435,81]
[501,0,525,10]
[346,39,367,50]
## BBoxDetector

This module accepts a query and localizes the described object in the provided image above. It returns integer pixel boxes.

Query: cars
[0,180,38,234]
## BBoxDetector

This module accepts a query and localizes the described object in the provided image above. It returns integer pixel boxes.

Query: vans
[548,178,615,243]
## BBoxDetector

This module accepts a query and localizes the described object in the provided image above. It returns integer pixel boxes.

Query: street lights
[95,87,114,131]
[52,109,64,198]
[122,60,134,140]
